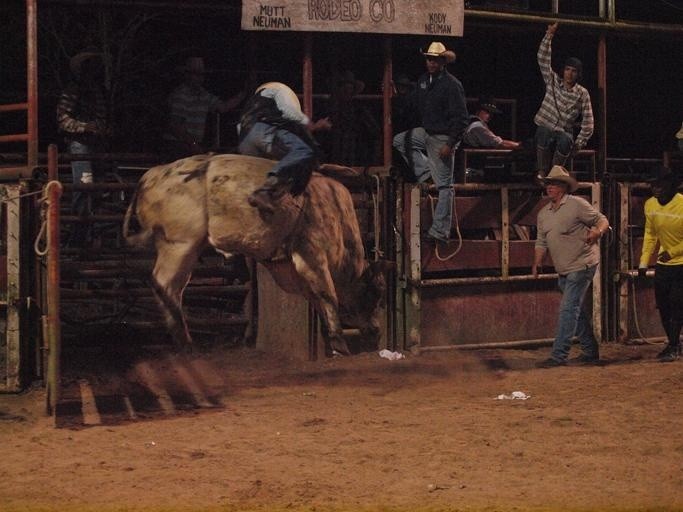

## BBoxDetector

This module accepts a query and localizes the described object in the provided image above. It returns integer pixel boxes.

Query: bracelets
[598,227,604,240]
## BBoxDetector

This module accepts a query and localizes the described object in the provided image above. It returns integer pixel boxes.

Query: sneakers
[535,359,564,368]
[659,345,680,362]
[569,354,599,364]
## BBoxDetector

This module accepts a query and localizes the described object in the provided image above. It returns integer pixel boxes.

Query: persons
[453,102,520,194]
[239,81,333,218]
[532,166,609,368]
[638,166,683,362]
[384,43,468,242]
[537,20,595,181]
[318,70,372,167]
[56,35,115,232]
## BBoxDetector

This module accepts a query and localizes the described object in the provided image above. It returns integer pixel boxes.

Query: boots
[247,175,296,215]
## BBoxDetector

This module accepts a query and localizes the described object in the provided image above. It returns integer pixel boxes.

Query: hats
[68,43,107,64]
[479,94,503,114]
[537,165,578,192]
[418,41,457,63]
[646,165,672,182]
[334,71,365,91]
